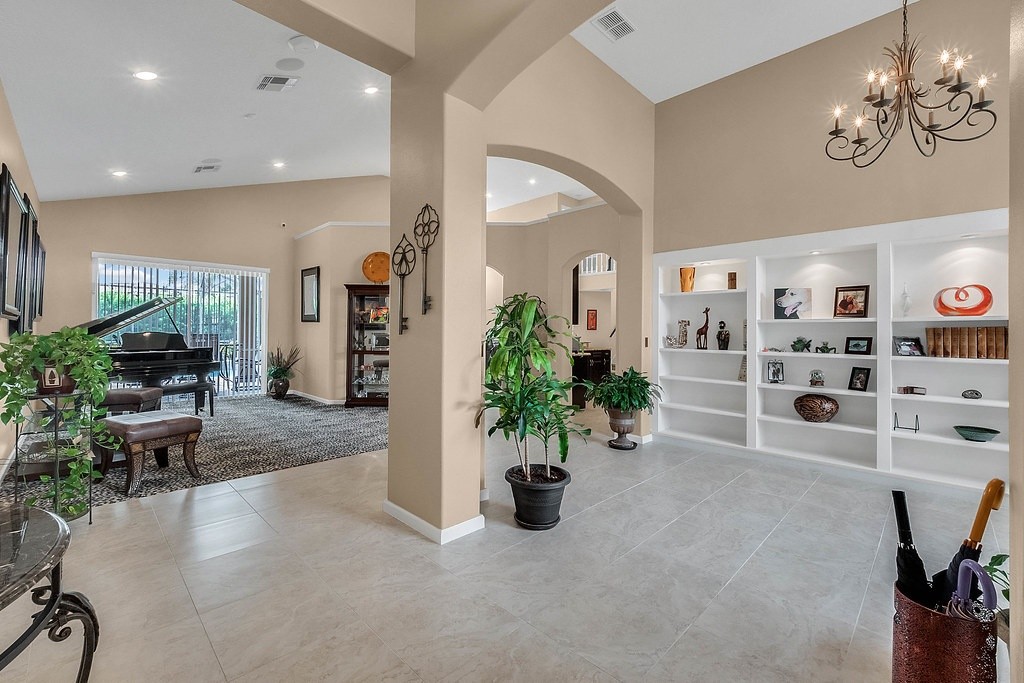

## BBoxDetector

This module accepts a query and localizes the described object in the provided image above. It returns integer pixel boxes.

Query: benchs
[143,380,214,417]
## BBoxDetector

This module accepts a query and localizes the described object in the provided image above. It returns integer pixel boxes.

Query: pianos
[72,295,221,417]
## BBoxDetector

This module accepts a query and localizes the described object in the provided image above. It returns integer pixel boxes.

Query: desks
[572,352,590,410]
[0,502,100,683]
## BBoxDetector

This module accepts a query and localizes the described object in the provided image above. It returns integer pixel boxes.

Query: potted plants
[0,326,124,515]
[267,343,305,400]
[584,365,664,451]
[474,291,594,531]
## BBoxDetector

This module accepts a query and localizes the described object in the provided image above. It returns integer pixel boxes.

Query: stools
[93,410,203,497]
[96,388,162,418]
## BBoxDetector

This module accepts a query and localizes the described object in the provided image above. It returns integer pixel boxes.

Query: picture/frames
[0,163,28,321]
[833,285,870,318]
[893,335,926,356]
[9,192,38,337]
[845,336,873,355]
[587,309,597,331]
[33,240,46,322]
[768,362,785,381]
[848,367,872,392]
[300,265,320,322]
[369,308,388,323]
[371,333,389,351]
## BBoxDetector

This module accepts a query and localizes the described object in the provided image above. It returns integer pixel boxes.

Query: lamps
[825,0,999,169]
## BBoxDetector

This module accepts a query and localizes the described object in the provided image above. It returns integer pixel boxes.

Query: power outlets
[612,364,615,370]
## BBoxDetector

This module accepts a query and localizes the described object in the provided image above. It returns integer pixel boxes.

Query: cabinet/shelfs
[657,255,750,450]
[890,227,1012,496]
[755,242,878,474]
[343,283,389,408]
[13,387,95,526]
[578,349,611,386]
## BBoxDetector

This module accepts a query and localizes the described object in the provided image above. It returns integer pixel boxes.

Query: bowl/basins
[953,426,1000,442]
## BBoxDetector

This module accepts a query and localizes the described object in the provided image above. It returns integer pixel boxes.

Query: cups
[680,267,696,292]
[728,272,737,290]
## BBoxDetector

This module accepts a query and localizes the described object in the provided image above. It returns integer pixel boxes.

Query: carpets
[0,392,388,511]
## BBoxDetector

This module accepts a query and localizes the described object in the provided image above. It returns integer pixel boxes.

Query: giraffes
[695,307,710,349]
